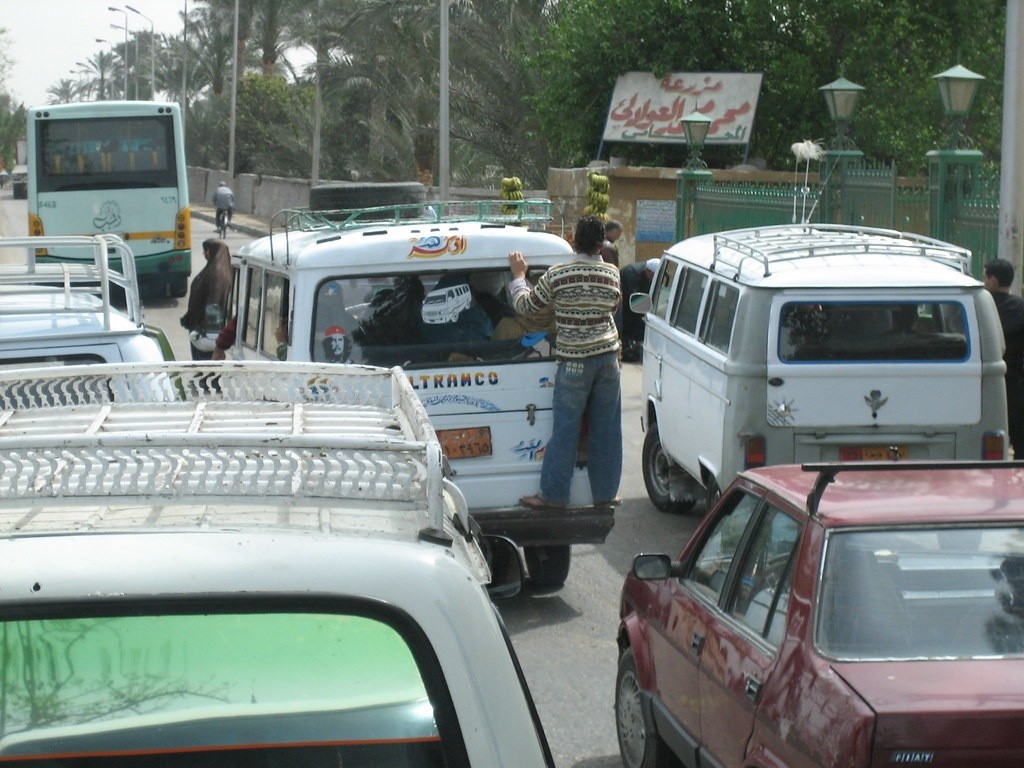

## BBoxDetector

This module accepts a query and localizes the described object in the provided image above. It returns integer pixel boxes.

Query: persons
[676,271,920,360]
[180,238,235,393]
[273,219,669,362]
[212,182,236,232]
[42,136,119,176]
[209,316,238,361]
[978,257,1024,463]
[508,216,625,513]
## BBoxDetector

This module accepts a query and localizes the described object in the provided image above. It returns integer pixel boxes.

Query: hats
[646,258,661,273]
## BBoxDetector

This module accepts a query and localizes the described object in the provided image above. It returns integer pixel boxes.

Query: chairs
[48,150,167,174]
[828,549,914,657]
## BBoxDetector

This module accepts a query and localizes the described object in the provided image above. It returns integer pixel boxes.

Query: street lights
[68,5,156,101]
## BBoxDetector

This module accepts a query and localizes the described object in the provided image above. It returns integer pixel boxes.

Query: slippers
[518,495,564,510]
[595,498,624,507]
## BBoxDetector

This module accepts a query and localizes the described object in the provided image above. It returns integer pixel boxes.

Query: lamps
[815,59,866,150]
[928,46,987,151]
[680,97,711,171]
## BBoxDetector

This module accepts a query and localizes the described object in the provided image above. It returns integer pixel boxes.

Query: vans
[629,222,1011,511]
[2,237,555,768]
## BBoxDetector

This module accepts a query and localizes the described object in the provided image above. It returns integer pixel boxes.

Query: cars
[616,452,1024,768]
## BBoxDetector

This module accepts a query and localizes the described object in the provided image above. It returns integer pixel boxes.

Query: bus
[27,100,192,301]
[202,196,619,599]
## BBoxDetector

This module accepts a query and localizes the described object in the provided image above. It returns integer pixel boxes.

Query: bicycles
[213,202,236,240]
